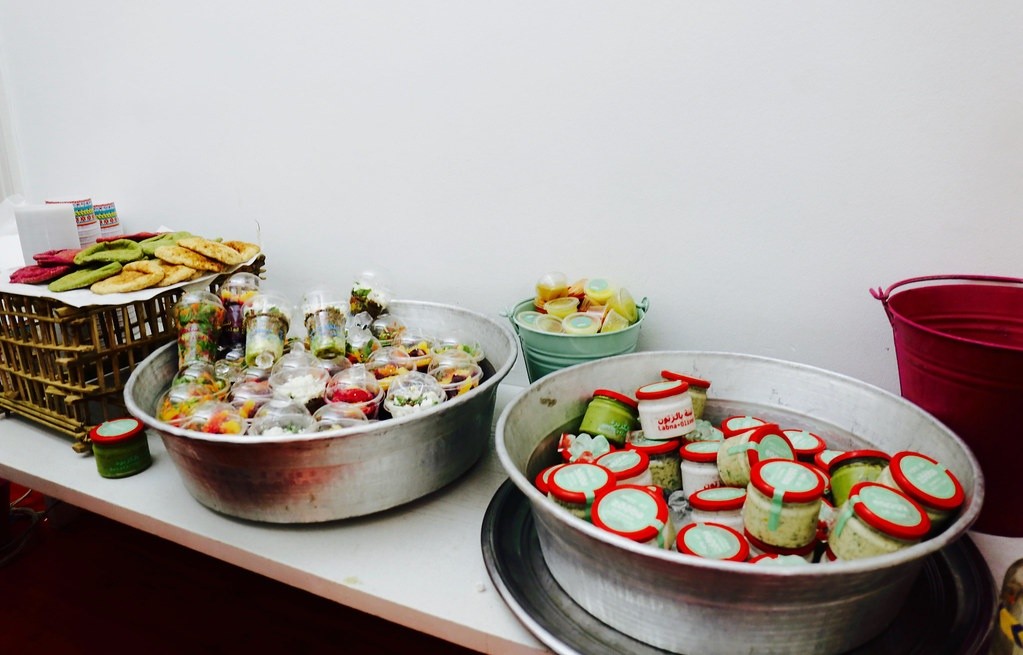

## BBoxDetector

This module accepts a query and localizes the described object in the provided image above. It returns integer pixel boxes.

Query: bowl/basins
[497,351,983,655]
[123,299,517,522]
[45,198,123,248]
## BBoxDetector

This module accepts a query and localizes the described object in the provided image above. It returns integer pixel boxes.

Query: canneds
[534,369,965,570]
[89,417,151,478]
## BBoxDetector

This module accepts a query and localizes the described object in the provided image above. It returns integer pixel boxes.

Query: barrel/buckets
[870,274,1023,537]
[501,293,648,385]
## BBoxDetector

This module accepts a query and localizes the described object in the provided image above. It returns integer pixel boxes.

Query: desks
[0,381,1023,655]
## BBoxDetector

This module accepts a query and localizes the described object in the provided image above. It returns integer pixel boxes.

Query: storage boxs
[0,254,268,451]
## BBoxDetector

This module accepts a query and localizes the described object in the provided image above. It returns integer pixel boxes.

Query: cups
[156,273,486,448]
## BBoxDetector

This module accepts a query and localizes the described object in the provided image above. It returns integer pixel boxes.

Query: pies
[9,231,262,293]
